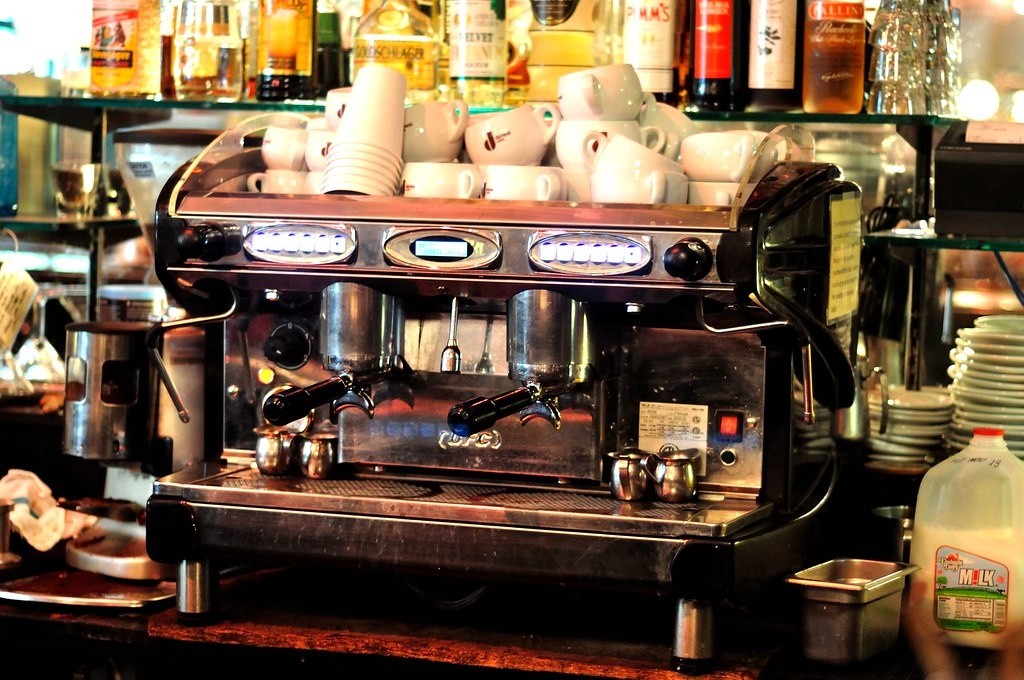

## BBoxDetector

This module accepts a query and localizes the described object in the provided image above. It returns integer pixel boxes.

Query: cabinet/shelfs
[0,96,1024,680]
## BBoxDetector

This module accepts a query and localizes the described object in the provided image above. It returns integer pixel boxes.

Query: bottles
[439,0,508,109]
[742,0,803,106]
[673,0,750,111]
[92,0,161,100]
[520,0,596,102]
[907,428,1024,651]
[350,0,439,103]
[596,0,677,106]
[804,0,865,113]
[257,0,315,100]
[316,0,346,89]
[73,47,91,98]
[170,0,242,103]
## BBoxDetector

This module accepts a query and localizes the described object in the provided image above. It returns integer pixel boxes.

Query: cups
[51,162,101,220]
[245,63,799,205]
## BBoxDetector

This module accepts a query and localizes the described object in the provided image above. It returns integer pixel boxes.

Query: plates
[791,315,1024,465]
[0,574,177,608]
